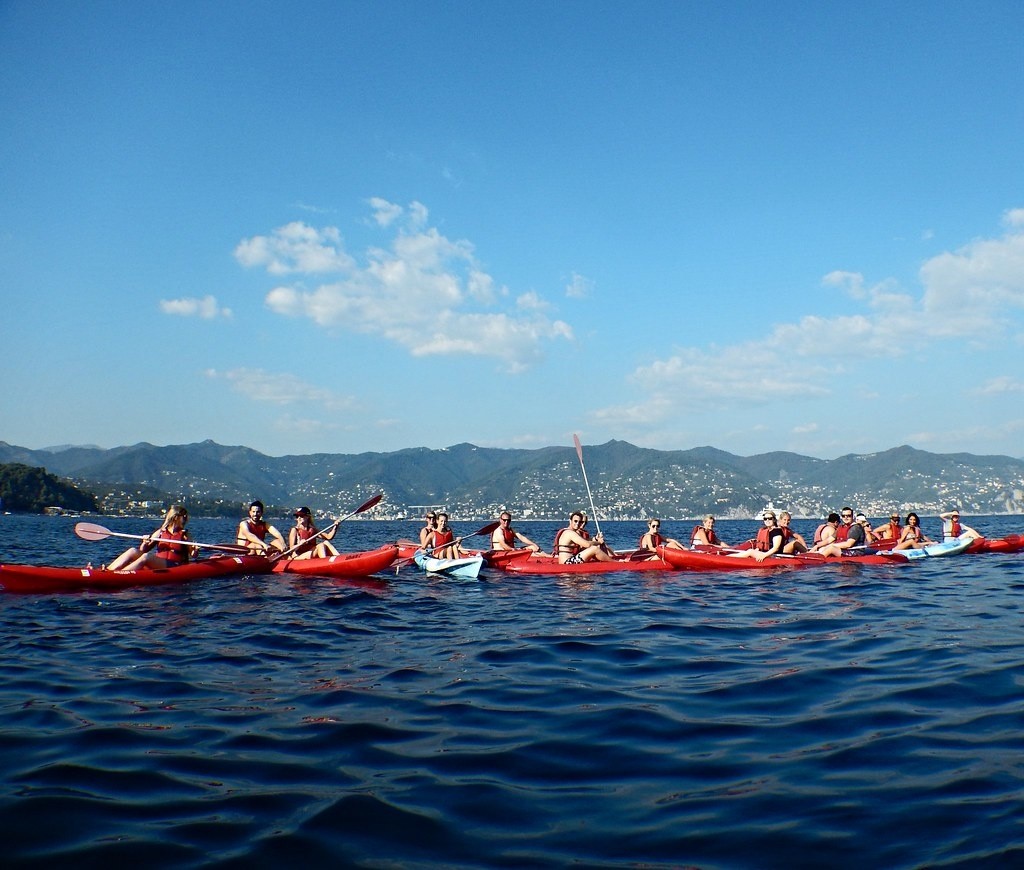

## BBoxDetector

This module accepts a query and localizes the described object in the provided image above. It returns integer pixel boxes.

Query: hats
[856,516,867,522]
[762,510,776,516]
[890,513,900,519]
[293,507,311,516]
[952,511,960,516]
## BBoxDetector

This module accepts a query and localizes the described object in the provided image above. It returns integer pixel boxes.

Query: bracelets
[458,542,460,544]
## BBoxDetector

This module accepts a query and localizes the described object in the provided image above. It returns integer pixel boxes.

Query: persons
[553,512,615,564]
[900,513,931,543]
[873,512,923,551]
[641,518,683,560]
[690,516,734,556]
[288,507,341,560]
[87,505,201,571]
[939,510,979,542]
[778,512,808,554]
[420,511,462,559]
[726,511,785,563]
[809,507,874,557]
[491,512,550,557]
[238,501,287,556]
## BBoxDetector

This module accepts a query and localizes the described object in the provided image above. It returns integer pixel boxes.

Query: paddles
[985,534,1020,544]
[249,546,291,552]
[629,550,658,562]
[388,521,501,568]
[74,522,251,555]
[695,544,826,565]
[269,495,383,564]
[394,542,422,549]
[572,433,601,536]
[847,538,898,551]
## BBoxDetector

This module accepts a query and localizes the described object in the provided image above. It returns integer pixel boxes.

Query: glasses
[175,515,187,521]
[501,518,512,522]
[763,517,774,520]
[652,524,660,528]
[574,520,582,524]
[426,516,436,520]
[296,516,305,518]
[841,515,852,518]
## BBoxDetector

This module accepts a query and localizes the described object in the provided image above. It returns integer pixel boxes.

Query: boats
[44,506,83,518]
[0,554,270,595]
[396,538,532,568]
[876,536,975,560]
[505,539,910,576]
[272,541,399,578]
[414,548,488,578]
[964,535,1024,554]
